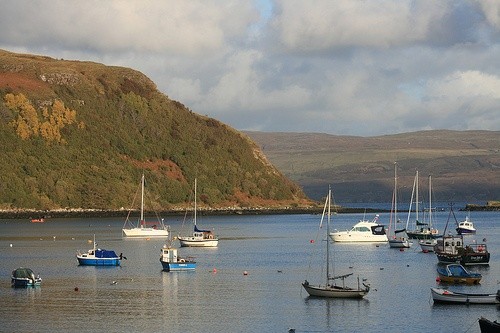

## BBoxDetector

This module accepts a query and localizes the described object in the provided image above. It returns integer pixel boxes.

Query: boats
[435,264,482,284]
[329,208,388,241]
[10,268,42,286]
[31,219,44,223]
[407,170,439,240]
[430,287,500,304]
[418,236,464,253]
[455,217,476,235]
[76,232,123,266]
[388,161,413,248]
[477,316,500,333]
[159,236,198,272]
[301,184,371,297]
[435,240,491,267]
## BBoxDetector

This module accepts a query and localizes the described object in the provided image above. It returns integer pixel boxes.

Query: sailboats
[121,171,170,235]
[177,177,218,247]
